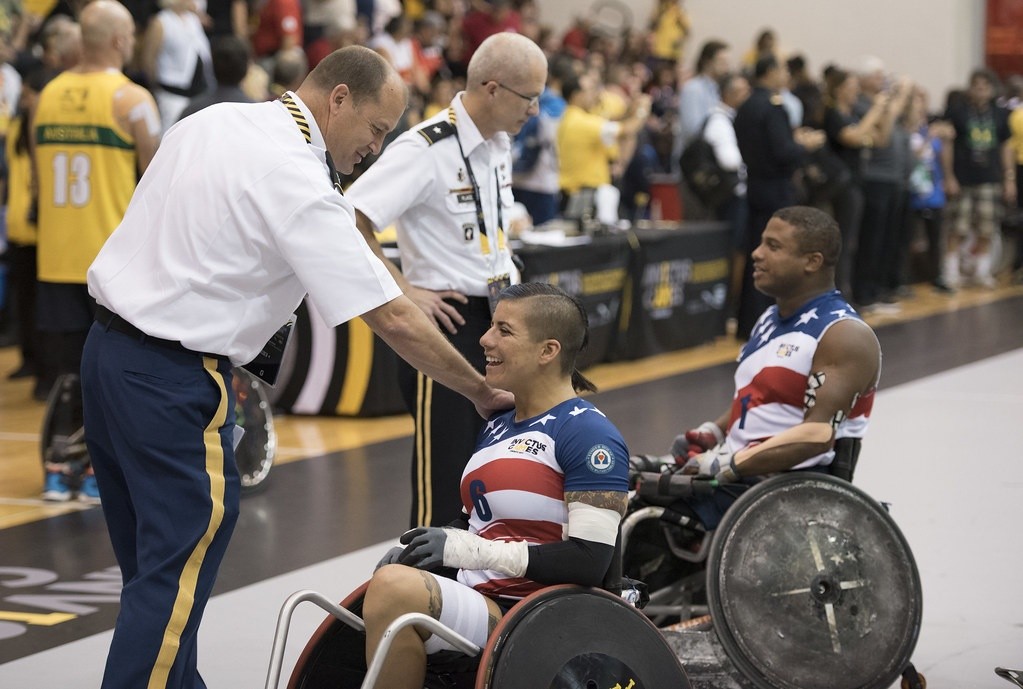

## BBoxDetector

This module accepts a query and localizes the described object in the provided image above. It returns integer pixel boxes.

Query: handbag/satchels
[678,112,745,213]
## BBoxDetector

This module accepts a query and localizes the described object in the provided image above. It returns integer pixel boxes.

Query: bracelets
[1005,169,1015,180]
[633,108,649,117]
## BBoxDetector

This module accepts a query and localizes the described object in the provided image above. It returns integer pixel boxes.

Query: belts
[93,306,219,371]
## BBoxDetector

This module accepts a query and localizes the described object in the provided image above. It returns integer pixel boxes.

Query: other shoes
[39,469,78,502]
[969,273,996,290]
[941,271,962,292]
[77,474,104,506]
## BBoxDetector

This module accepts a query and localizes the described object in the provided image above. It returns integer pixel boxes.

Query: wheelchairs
[265,436,925,689]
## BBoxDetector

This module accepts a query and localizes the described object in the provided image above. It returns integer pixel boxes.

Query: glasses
[482,79,543,107]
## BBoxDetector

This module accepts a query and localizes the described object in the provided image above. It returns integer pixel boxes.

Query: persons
[363,283,632,689]
[344,31,548,529]
[621,206,882,591]
[0,1,1023,378]
[28,0,161,500]
[733,53,826,341]
[940,69,1016,290]
[80,44,516,689]
[556,73,654,217]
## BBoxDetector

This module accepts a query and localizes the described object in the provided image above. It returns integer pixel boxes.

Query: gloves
[397,525,528,578]
[670,421,725,468]
[673,451,742,483]
[373,546,404,572]
